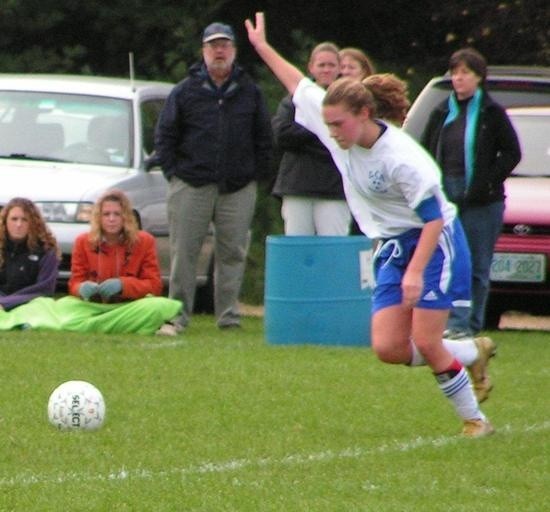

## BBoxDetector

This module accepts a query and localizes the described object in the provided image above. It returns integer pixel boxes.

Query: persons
[420,48,522,339]
[68,189,163,304]
[244,11,496,439]
[269,42,373,237]
[0,196,62,311]
[154,22,277,336]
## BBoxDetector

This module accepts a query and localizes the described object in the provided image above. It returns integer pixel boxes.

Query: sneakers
[155,321,184,336]
[467,336,498,404]
[460,416,496,441]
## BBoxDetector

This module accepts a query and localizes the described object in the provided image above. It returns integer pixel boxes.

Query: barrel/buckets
[264,234,376,346]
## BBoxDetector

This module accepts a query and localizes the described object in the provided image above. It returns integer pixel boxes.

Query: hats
[202,21,235,44]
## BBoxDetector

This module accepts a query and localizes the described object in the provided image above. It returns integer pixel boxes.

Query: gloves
[78,279,99,299]
[99,277,122,299]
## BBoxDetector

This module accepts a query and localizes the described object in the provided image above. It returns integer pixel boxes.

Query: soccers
[47,381,105,430]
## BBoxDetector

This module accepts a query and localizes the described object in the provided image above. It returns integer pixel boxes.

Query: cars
[1,52,251,312]
[401,65,550,329]
